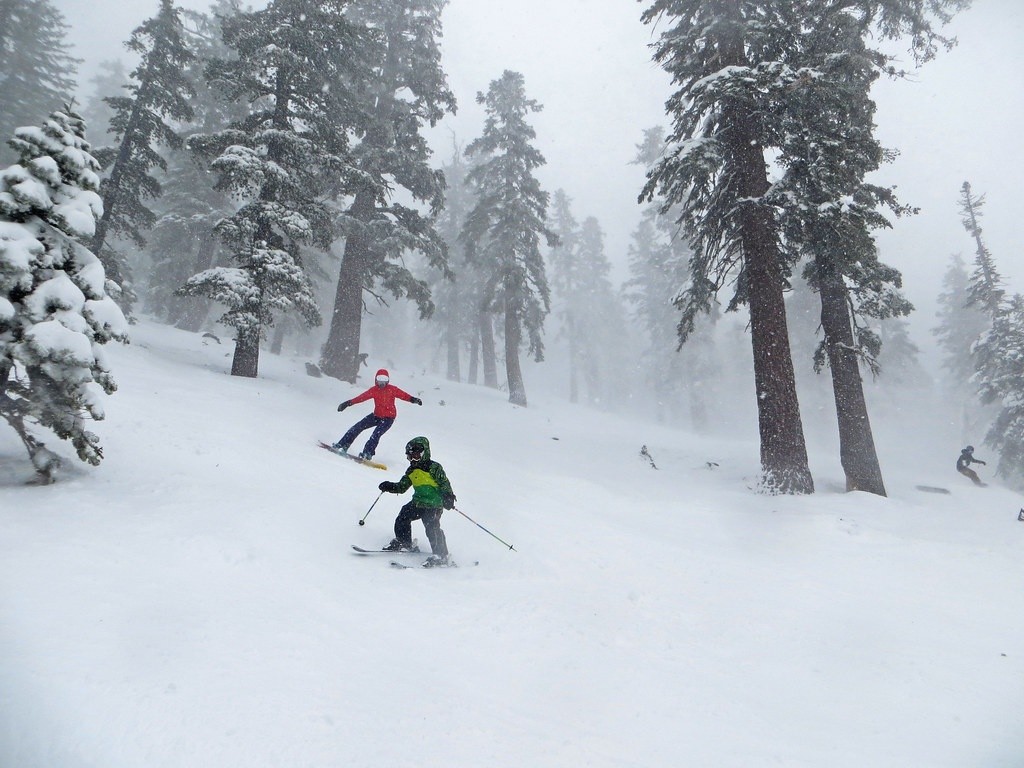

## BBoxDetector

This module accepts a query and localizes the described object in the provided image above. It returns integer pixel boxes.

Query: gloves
[378,480,395,493]
[337,400,353,412]
[410,396,422,406]
[443,495,456,511]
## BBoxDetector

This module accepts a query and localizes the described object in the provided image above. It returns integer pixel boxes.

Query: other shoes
[332,442,348,453]
[422,552,453,568]
[359,451,373,461]
[382,537,413,551]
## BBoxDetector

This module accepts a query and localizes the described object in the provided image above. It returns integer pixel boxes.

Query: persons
[333,370,422,461]
[359,353,369,371]
[378,435,456,566]
[957,445,987,487]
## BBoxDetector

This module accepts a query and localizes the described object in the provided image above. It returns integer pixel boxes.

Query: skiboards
[351,544,480,569]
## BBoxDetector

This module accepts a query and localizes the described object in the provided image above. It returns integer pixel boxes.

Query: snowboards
[314,439,387,471]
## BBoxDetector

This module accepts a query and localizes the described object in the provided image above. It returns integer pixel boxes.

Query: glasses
[405,443,424,453]
[377,374,389,382]
